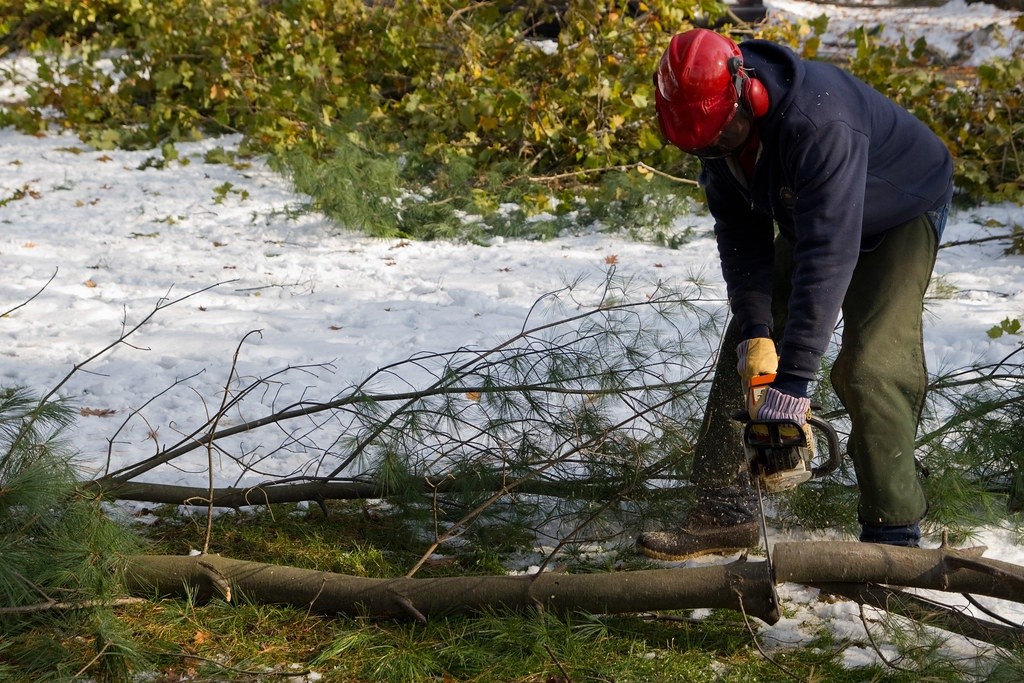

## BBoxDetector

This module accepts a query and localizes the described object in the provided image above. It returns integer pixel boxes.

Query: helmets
[653,26,746,151]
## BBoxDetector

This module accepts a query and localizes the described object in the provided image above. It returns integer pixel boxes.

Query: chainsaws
[729,374,840,618]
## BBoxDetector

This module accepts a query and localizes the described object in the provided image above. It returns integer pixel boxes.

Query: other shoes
[636,504,759,558]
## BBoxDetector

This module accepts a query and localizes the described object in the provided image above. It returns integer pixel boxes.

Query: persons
[636,28,952,592]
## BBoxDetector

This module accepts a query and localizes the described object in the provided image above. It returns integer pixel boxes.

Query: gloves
[736,336,779,414]
[749,383,815,461]
